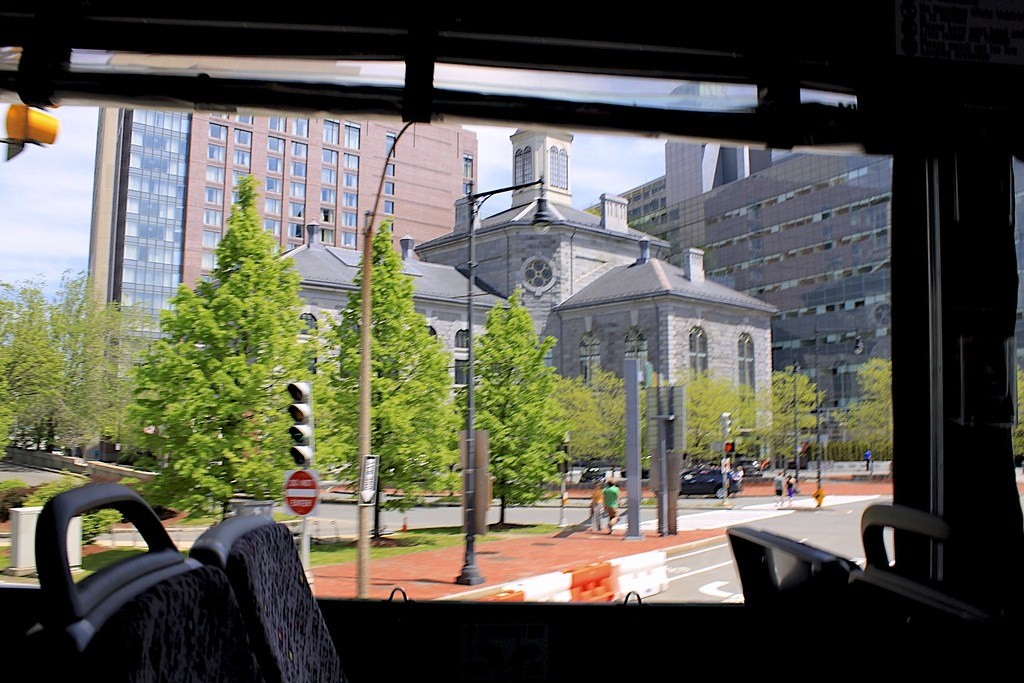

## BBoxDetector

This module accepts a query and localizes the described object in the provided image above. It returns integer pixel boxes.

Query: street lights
[814,322,864,504]
[458,178,564,582]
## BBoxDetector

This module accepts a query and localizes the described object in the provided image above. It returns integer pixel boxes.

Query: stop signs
[284,471,318,517]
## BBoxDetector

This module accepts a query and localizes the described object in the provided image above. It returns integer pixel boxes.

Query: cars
[679,470,740,498]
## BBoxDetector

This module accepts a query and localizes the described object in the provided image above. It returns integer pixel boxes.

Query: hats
[607,478,615,485]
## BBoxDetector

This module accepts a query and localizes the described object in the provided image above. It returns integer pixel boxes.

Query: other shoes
[607,523,612,533]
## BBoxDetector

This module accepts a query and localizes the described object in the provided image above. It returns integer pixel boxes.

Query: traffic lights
[725,442,734,451]
[722,412,732,434]
[6,99,58,162]
[288,381,315,467]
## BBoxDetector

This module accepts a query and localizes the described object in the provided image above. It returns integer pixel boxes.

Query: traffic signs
[357,454,380,506]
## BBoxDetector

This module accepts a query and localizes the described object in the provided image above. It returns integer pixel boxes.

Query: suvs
[733,459,761,477]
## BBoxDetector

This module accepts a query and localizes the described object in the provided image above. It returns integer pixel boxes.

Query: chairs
[25,482,349,683]
[724,503,1024,683]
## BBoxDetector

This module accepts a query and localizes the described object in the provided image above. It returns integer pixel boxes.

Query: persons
[864,449,874,472]
[590,478,621,535]
[725,465,746,497]
[775,473,794,507]
